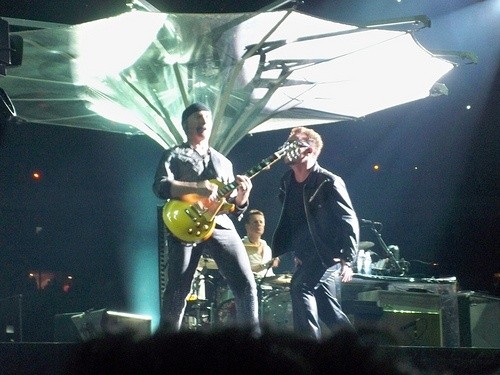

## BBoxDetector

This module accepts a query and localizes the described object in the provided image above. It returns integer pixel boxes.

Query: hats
[182,103,209,121]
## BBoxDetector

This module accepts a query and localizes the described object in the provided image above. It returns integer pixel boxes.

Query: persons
[269,125,360,340]
[153,103,261,337]
[220,208,282,307]
[48,323,423,375]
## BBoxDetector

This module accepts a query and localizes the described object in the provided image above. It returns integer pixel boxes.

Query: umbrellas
[0,0,482,158]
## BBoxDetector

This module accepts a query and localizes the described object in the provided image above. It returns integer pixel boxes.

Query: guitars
[161,140,299,246]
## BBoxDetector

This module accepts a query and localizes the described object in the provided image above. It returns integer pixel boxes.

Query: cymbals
[352,240,377,251]
[258,274,294,286]
[197,256,220,270]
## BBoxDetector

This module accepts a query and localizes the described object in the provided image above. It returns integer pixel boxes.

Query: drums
[215,284,247,330]
[345,251,373,277]
[259,286,300,339]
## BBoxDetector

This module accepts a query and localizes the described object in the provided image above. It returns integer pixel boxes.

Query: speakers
[357,291,460,348]
[342,300,382,346]
[461,292,500,348]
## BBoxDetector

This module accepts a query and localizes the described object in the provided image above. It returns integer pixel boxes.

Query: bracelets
[345,263,352,268]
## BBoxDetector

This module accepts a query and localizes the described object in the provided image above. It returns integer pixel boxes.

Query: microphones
[359,218,383,228]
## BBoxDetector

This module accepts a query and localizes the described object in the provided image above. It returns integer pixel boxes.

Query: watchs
[263,262,268,268]
